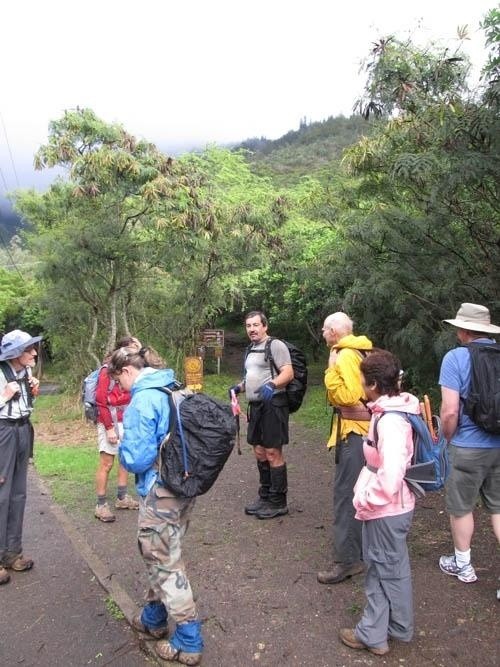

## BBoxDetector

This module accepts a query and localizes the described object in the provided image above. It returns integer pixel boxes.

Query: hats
[0,328,43,360]
[443,303,500,336]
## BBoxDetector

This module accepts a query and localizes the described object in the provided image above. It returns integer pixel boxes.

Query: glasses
[25,347,37,355]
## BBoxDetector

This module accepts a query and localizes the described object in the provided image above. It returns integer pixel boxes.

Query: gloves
[227,384,239,399]
[254,381,276,401]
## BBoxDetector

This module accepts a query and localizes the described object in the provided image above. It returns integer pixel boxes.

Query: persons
[229,312,308,521]
[92,338,144,522]
[438,302,499,599]
[0,329,38,586]
[338,349,420,655]
[109,344,203,662]
[316,311,380,582]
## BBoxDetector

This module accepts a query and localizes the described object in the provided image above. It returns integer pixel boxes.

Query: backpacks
[454,339,500,435]
[81,366,114,422]
[363,412,446,493]
[248,338,307,413]
[134,383,233,497]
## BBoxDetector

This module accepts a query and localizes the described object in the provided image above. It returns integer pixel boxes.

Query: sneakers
[94,503,117,523]
[338,626,389,655]
[243,501,263,515]
[153,634,201,666]
[316,561,362,584]
[0,567,10,584]
[7,556,35,572]
[439,554,477,584]
[115,495,139,511]
[131,612,169,637]
[253,506,289,519]
